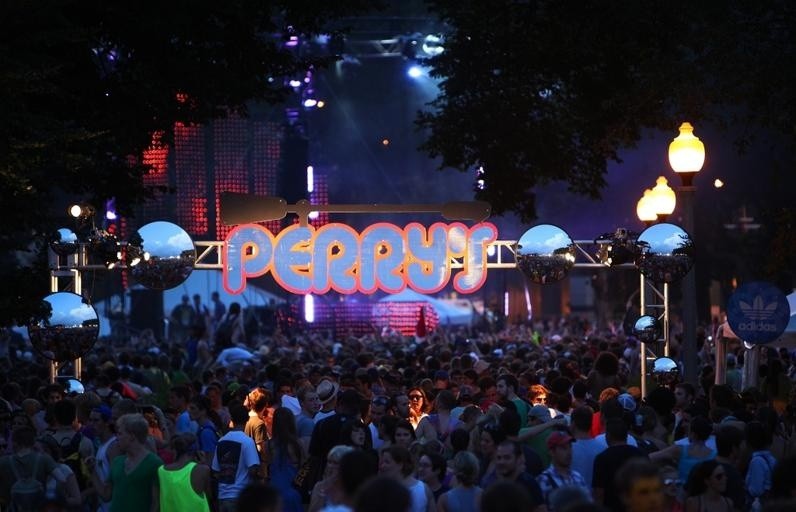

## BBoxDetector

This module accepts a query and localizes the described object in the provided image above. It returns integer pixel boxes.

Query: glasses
[374,395,423,404]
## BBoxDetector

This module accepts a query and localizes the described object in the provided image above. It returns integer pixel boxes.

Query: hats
[316,380,339,404]
[528,405,550,423]
[473,360,490,373]
[94,406,112,422]
[457,451,479,476]
[547,432,576,448]
[618,393,636,412]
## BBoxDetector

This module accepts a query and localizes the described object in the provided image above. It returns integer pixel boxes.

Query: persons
[0,277,794,511]
[643,252,687,278]
[515,252,576,281]
[125,259,196,286]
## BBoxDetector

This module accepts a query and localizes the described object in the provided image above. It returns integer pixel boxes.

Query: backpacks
[10,431,91,512]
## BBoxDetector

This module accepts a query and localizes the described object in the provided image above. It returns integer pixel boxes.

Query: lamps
[69,203,98,235]
[590,222,629,273]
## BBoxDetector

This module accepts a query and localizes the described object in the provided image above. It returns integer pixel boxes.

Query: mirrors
[631,310,666,347]
[648,351,682,388]
[636,223,695,281]
[125,219,196,291]
[50,228,79,256]
[514,223,575,285]
[56,374,90,398]
[26,292,100,362]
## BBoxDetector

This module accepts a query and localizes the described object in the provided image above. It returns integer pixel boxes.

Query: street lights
[668,122,705,405]
[636,188,657,228]
[650,172,677,225]
